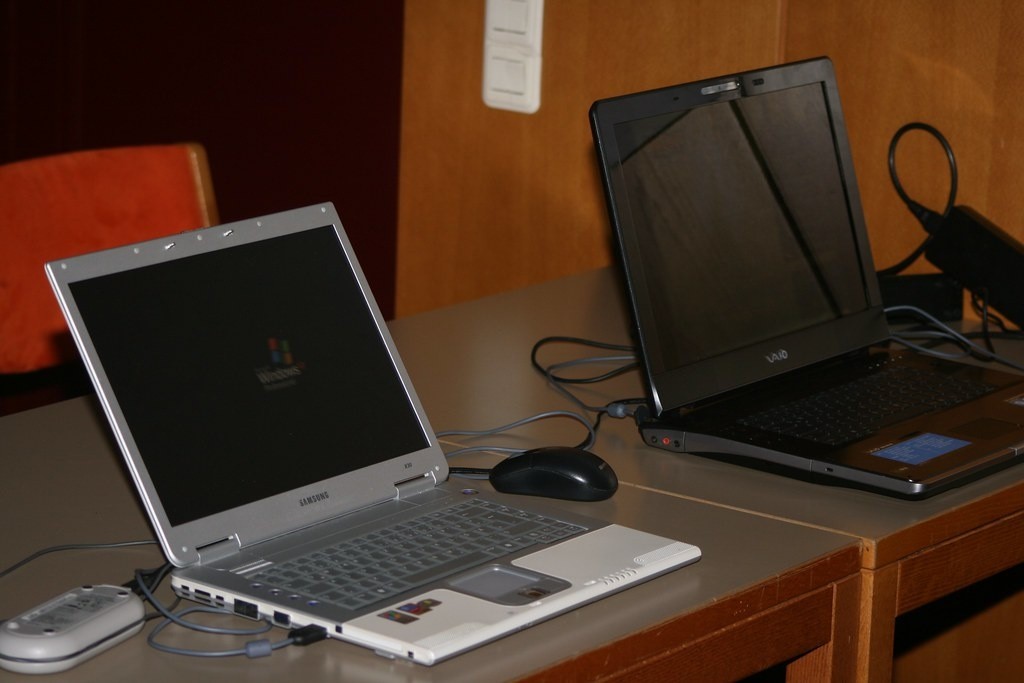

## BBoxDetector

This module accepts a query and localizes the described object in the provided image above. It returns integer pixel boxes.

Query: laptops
[44,201,702,667]
[588,56,1024,502]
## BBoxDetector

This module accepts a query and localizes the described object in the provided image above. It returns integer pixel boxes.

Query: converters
[925,203,1024,331]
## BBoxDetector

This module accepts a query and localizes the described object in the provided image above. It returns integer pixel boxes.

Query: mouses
[488,445,619,502]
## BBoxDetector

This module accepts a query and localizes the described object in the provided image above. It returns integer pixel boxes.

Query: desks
[388,262,1024,683]
[0,390,865,683]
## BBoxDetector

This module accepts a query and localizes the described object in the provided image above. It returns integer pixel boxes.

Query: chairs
[0,143,219,375]
